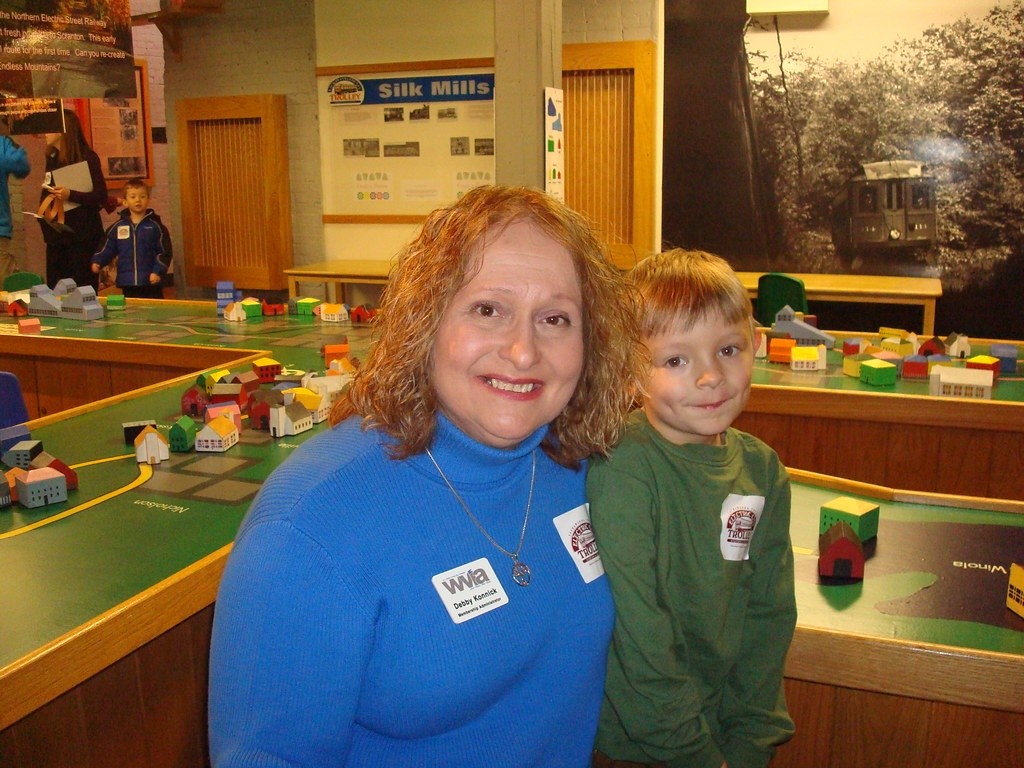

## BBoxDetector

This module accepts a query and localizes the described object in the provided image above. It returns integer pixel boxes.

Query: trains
[825,161,936,266]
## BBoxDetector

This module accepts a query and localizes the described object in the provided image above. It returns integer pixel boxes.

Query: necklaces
[422,441,536,585]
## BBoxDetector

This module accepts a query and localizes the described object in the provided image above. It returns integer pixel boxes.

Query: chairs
[4,272,42,292]
[757,274,809,327]
[0,371,29,429]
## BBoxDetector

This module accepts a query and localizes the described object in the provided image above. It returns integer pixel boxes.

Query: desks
[734,271,943,336]
[729,327,1023,503]
[771,468,1024,768]
[0,293,383,768]
[283,258,400,304]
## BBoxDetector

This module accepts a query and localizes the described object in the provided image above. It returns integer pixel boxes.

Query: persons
[0,95,171,299]
[206,186,652,768]
[584,248,796,768]
